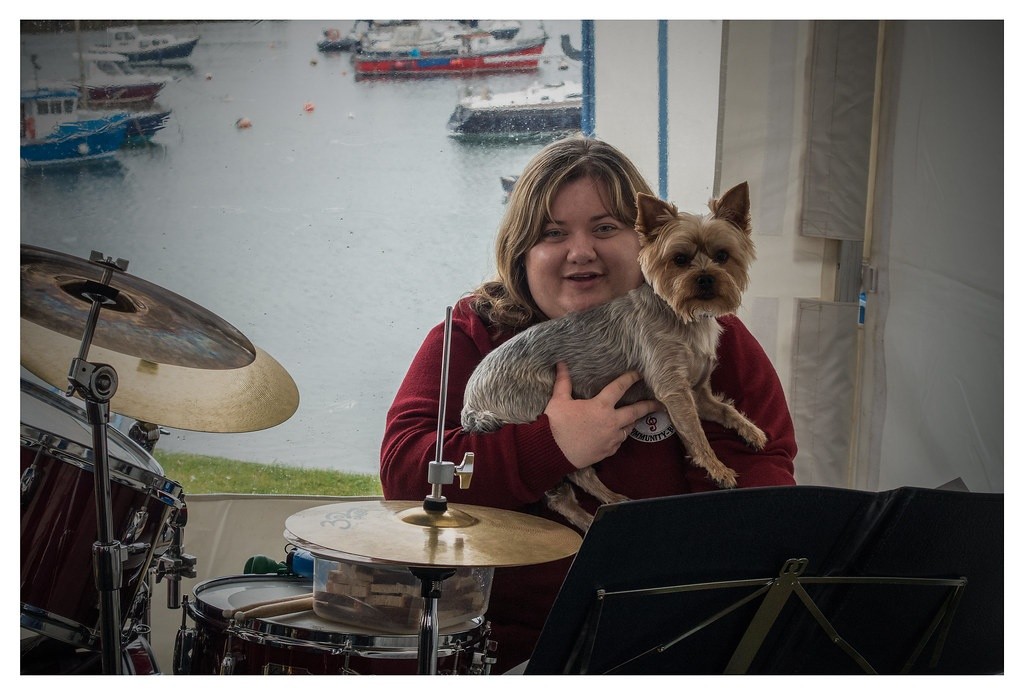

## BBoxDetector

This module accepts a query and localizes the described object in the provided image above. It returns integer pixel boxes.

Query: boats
[317,19,519,52]
[447,80,583,143]
[21,53,166,105]
[88,24,199,69]
[21,54,142,167]
[351,20,550,75]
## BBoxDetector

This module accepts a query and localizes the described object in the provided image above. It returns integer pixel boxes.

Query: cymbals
[281,498,585,569]
[21,316,301,434]
[20,244,301,371]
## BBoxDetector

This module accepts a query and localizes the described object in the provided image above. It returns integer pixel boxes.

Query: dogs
[460,180,768,534]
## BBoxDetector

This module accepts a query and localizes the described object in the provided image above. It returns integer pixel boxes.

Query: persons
[379,139,797,540]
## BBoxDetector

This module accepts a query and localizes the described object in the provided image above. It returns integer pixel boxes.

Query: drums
[20,379,195,655]
[20,620,160,678]
[174,574,500,676]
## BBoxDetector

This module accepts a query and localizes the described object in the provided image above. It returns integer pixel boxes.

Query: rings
[622,430,627,441]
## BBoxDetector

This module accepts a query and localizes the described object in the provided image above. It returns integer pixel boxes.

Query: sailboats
[32,21,172,145]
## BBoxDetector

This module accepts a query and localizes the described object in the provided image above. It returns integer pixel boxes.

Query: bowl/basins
[313,552,495,633]
